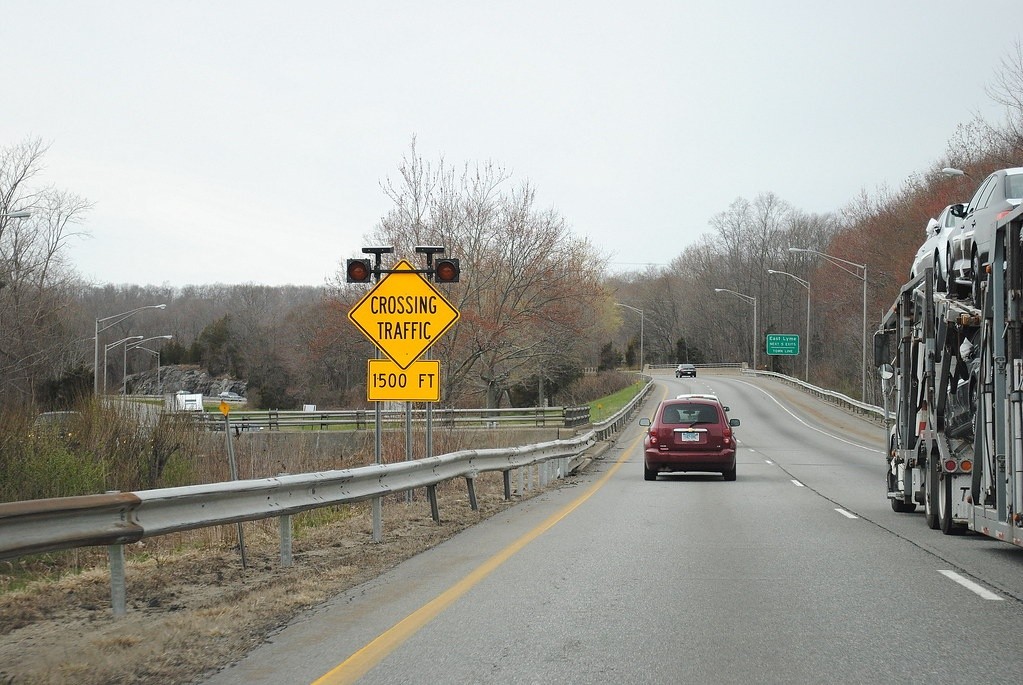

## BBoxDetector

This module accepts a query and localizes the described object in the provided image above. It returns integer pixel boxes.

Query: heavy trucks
[873,168,1023,546]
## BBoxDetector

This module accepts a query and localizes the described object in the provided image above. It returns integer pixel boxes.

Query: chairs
[663,406,680,424]
[698,406,718,424]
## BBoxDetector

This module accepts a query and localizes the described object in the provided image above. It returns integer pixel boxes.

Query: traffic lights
[346,258,372,283]
[435,259,460,284]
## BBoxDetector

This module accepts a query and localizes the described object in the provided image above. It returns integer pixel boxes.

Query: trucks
[169,390,203,418]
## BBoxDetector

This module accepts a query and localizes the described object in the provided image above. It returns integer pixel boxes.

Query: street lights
[135,346,160,396]
[768,270,810,381]
[104,336,143,398]
[715,288,757,372]
[94,303,166,399]
[788,246,867,402]
[123,335,173,398]
[613,302,643,371]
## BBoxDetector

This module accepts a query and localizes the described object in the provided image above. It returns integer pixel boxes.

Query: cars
[675,364,696,378]
[219,392,242,400]
[676,394,730,417]
[30,410,86,448]
[639,397,740,481]
